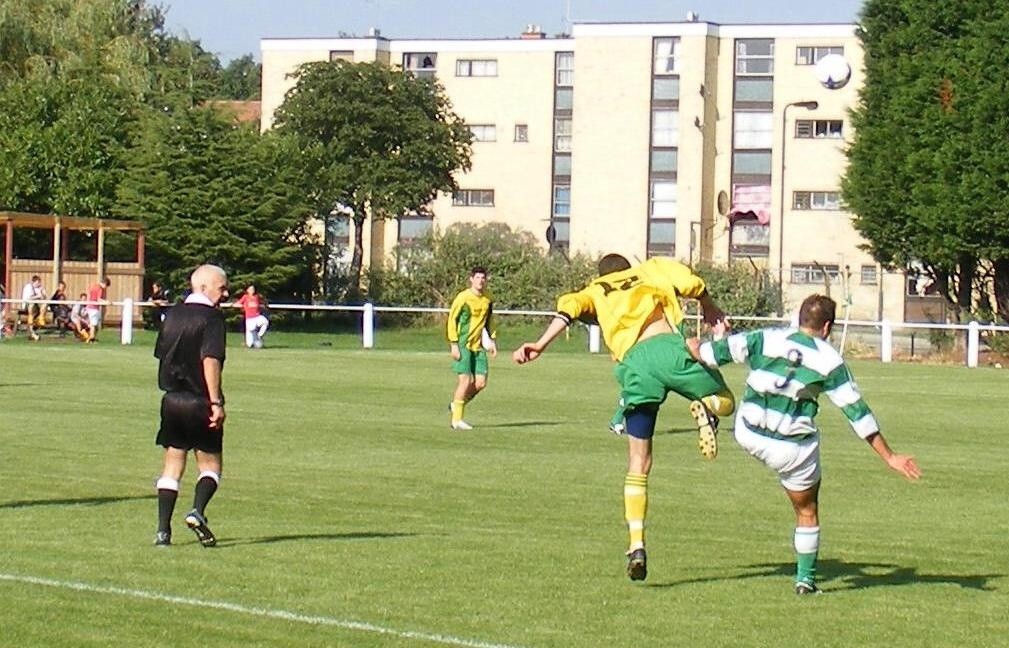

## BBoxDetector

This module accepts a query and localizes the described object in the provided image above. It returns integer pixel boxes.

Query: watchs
[211,399,224,406]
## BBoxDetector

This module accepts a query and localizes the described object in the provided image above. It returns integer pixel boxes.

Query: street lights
[778,101,818,311]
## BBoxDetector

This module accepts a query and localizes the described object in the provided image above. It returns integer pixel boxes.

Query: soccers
[813,53,851,90]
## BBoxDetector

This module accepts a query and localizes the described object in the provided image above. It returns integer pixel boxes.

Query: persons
[234,283,269,348]
[445,267,497,430]
[685,292,920,594]
[22,275,110,345]
[153,263,229,547]
[513,253,725,581]
[147,269,192,329]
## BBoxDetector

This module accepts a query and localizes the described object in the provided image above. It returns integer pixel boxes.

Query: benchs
[12,311,58,336]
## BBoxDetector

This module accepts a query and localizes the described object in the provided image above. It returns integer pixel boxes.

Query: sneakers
[154,531,171,546]
[796,583,822,596]
[185,508,217,547]
[625,549,647,581]
[608,422,627,437]
[688,399,719,460]
[451,421,473,431]
[449,403,454,413]
